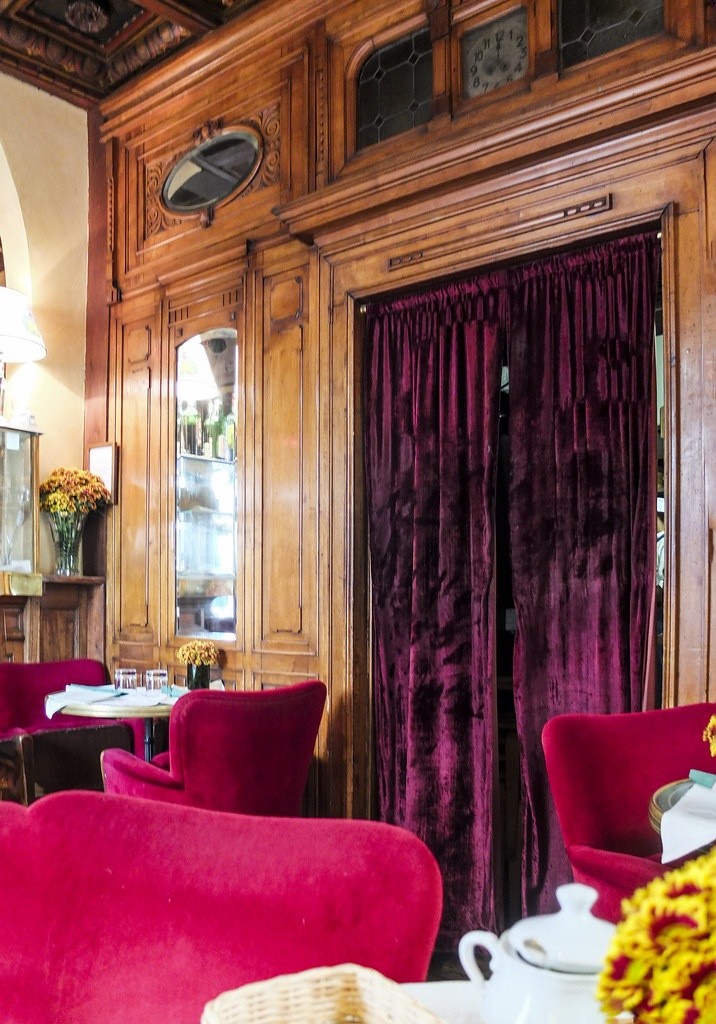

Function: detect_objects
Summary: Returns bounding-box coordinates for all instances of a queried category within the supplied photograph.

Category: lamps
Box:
[0,285,49,413]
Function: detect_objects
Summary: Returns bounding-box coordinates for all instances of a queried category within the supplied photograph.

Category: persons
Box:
[656,491,667,588]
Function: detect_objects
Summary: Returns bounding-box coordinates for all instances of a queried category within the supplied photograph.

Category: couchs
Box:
[0,658,149,803]
[540,699,716,928]
[0,789,443,1024]
[100,680,328,819]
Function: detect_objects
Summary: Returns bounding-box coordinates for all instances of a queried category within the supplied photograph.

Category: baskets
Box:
[202,963,451,1023]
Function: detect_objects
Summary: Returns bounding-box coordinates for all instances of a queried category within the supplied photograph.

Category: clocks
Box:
[449,0,538,120]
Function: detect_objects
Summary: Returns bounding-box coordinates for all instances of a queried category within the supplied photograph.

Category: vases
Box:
[186,663,210,689]
[47,510,88,577]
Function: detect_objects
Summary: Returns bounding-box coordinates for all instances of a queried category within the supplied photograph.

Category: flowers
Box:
[177,640,221,666]
[38,466,113,568]
[597,844,716,1024]
[703,714,716,757]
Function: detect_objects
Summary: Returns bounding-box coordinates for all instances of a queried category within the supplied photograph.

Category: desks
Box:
[648,779,716,858]
[43,689,172,760]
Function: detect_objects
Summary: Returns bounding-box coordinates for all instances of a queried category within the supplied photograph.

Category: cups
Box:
[457,882,625,1024]
[145,669,168,699]
[114,666,137,694]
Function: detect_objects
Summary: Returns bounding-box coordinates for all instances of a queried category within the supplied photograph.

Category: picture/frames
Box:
[86,443,119,506]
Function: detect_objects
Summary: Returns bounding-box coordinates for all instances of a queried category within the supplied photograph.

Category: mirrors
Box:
[173,325,240,642]
[156,123,263,218]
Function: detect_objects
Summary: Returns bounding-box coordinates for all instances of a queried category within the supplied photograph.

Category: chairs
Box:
[13,720,135,806]
